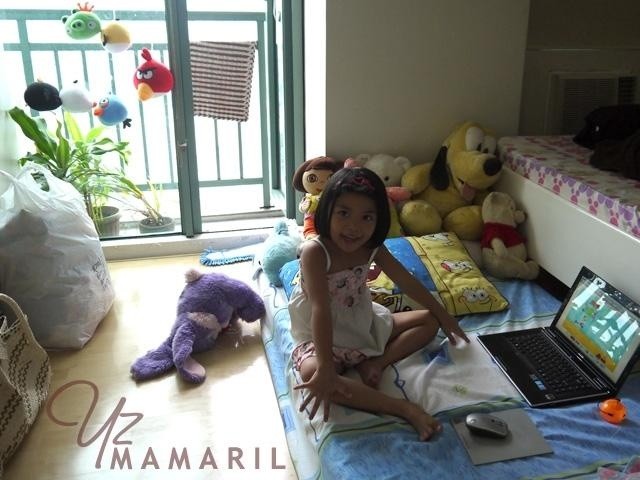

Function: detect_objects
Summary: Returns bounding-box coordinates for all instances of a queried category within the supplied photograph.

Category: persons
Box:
[251,120,540,293]
[286,165,469,441]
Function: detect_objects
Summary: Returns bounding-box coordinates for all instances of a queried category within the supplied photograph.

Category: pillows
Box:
[281,232,512,322]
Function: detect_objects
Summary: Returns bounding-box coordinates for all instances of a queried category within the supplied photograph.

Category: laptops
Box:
[476,265,640,408]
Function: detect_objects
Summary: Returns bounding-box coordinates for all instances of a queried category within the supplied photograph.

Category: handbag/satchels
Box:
[0,293,53,478]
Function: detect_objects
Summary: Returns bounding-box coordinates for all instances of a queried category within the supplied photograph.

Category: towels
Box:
[188,40,257,123]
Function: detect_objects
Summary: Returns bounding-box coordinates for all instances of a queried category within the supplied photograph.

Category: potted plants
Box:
[9,106,175,238]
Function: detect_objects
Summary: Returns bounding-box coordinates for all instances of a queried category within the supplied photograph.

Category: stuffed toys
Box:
[24,79,62,112]
[61,2,101,42]
[133,47,174,101]
[93,90,133,128]
[595,396,627,425]
[128,269,265,387]
[100,21,132,52]
[58,81,97,114]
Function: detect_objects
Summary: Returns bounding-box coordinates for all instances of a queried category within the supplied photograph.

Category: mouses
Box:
[465,413,508,439]
